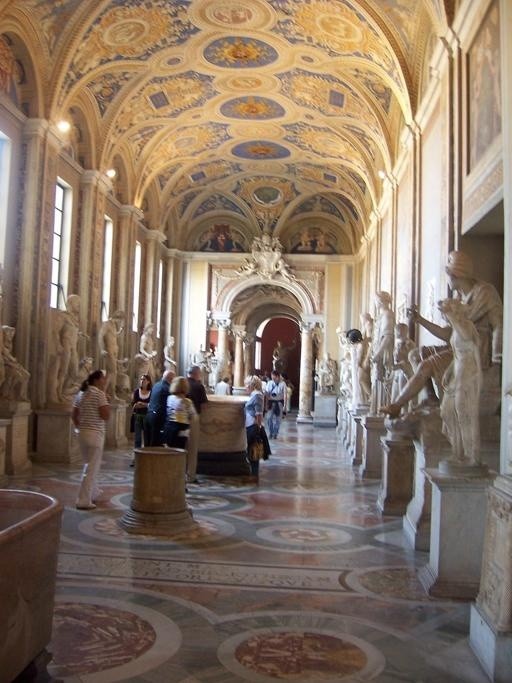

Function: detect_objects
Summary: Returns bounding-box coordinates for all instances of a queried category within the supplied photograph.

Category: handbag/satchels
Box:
[248,438,265,462]
[130,413,136,433]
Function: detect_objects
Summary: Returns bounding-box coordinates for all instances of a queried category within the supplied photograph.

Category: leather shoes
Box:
[192,478,200,484]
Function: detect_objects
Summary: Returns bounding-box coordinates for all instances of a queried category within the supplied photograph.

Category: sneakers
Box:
[273,433,278,439]
[268,433,273,440]
[77,503,97,509]
[185,488,190,494]
[129,459,135,467]
[92,489,104,503]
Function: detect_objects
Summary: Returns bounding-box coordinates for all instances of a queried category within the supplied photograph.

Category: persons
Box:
[317,247,502,467]
[70,369,109,510]
[272,337,297,373]
[0,294,213,413]
[129,367,294,483]
